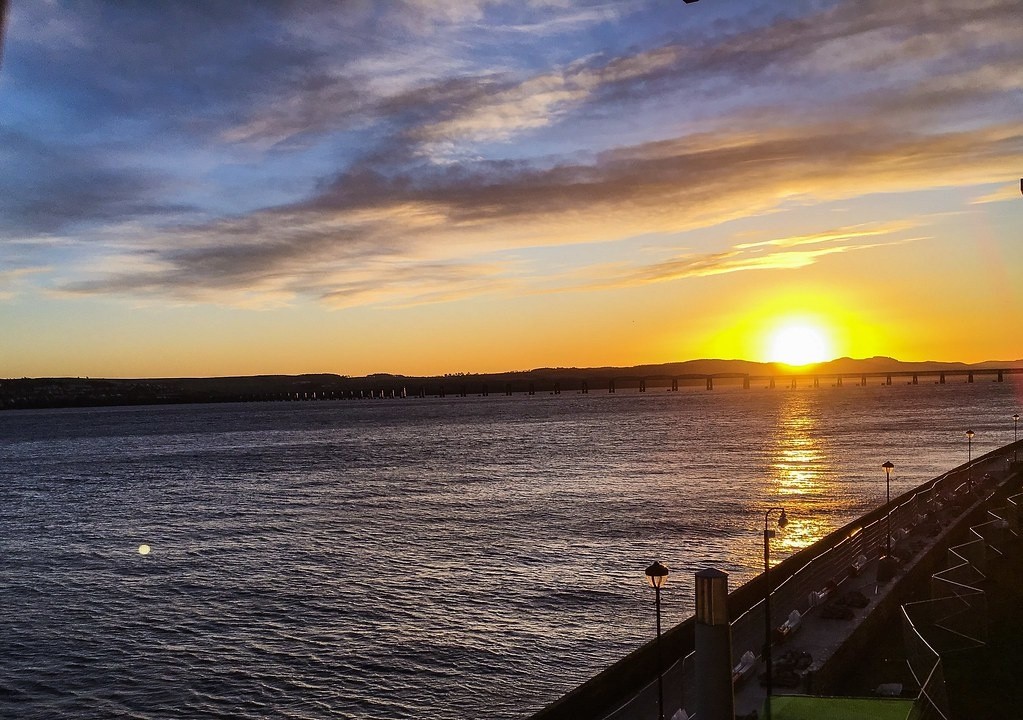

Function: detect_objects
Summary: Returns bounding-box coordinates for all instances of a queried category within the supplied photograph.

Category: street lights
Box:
[881,460,896,558]
[1011,413,1020,462]
[964,430,975,495]
[644,560,668,720]
[763,506,790,716]
